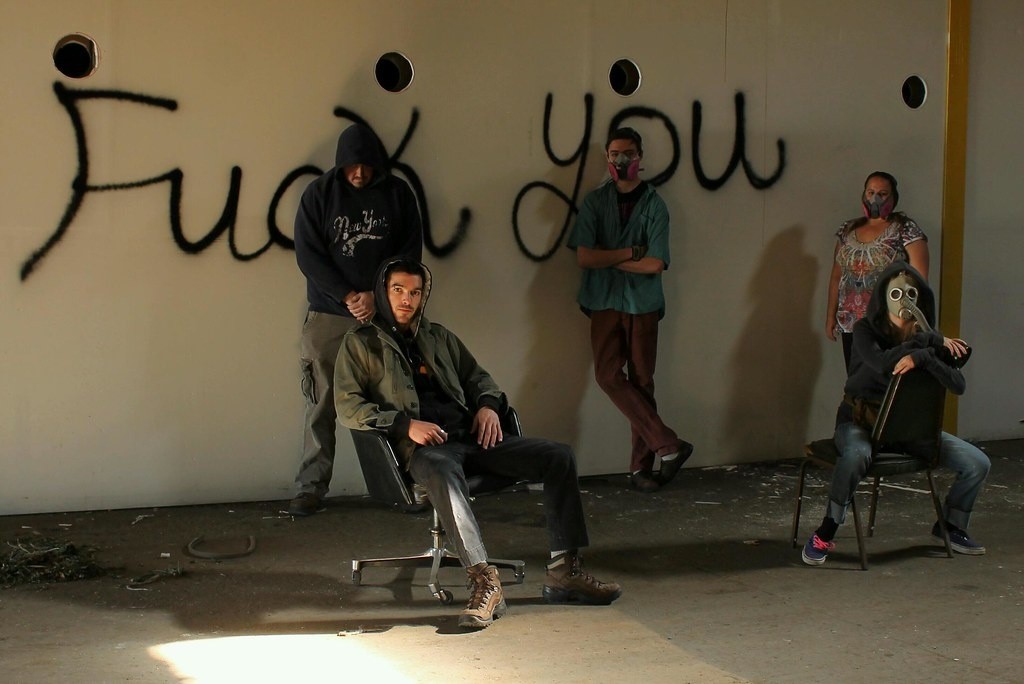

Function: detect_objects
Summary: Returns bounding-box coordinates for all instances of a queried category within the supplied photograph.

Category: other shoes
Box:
[628,470,660,493]
[657,440,694,486]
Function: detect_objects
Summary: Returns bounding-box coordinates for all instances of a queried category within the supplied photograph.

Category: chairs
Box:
[789,367,955,572]
[350,406,526,607]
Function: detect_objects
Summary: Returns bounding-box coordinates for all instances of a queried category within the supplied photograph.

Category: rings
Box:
[439,431,444,435]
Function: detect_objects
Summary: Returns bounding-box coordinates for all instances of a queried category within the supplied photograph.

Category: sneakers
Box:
[931,522,986,554]
[802,531,835,565]
[288,493,322,515]
[457,563,508,627]
[542,552,623,604]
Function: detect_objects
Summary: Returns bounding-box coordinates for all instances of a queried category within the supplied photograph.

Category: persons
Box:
[565,126,695,493]
[825,173,929,380]
[284,123,427,517]
[802,261,990,564]
[334,254,620,627]
